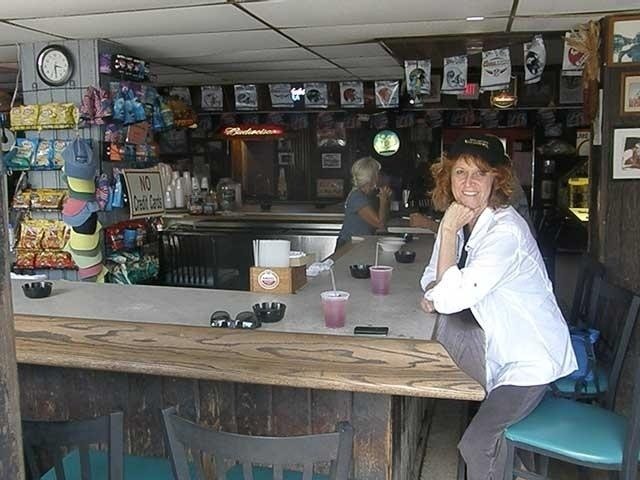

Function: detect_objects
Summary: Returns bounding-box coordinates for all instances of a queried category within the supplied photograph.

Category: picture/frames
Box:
[610,125,640,183]
[319,152,342,170]
[617,69,639,118]
[605,17,640,67]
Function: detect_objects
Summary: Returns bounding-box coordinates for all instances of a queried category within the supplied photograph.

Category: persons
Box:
[408,185,538,239]
[334,157,393,253]
[418,128,580,480]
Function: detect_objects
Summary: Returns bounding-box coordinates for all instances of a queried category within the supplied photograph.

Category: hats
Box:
[449,134,504,164]
[62,138,108,283]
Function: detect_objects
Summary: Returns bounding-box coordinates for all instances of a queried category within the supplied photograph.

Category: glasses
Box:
[210,311,261,328]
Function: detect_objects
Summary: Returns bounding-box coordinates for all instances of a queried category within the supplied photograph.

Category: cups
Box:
[369,265,395,293]
[320,291,350,330]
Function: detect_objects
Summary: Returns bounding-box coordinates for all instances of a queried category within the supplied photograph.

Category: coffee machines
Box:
[398,183,421,217]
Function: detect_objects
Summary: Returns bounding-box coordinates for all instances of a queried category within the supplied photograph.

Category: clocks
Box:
[38,43,74,86]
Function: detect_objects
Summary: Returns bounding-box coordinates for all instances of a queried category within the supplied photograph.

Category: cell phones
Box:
[354,326,388,334]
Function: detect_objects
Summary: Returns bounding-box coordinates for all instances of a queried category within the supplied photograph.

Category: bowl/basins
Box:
[231,312,260,329]
[165,163,236,217]
[376,236,416,263]
[210,309,232,328]
[23,282,53,297]
[348,262,370,278]
[252,301,286,322]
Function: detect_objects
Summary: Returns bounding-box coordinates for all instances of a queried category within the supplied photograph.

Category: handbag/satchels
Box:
[570,329,594,381]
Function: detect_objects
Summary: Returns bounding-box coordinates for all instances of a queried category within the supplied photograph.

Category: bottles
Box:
[278,165,288,201]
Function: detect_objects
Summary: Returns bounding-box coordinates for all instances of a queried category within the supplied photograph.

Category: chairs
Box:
[506,360,640,480]
[553,253,603,330]
[160,403,356,480]
[153,230,239,291]
[550,274,639,410]
[20,410,191,479]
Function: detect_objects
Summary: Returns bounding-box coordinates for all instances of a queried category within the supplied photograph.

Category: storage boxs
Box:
[248,263,306,293]
